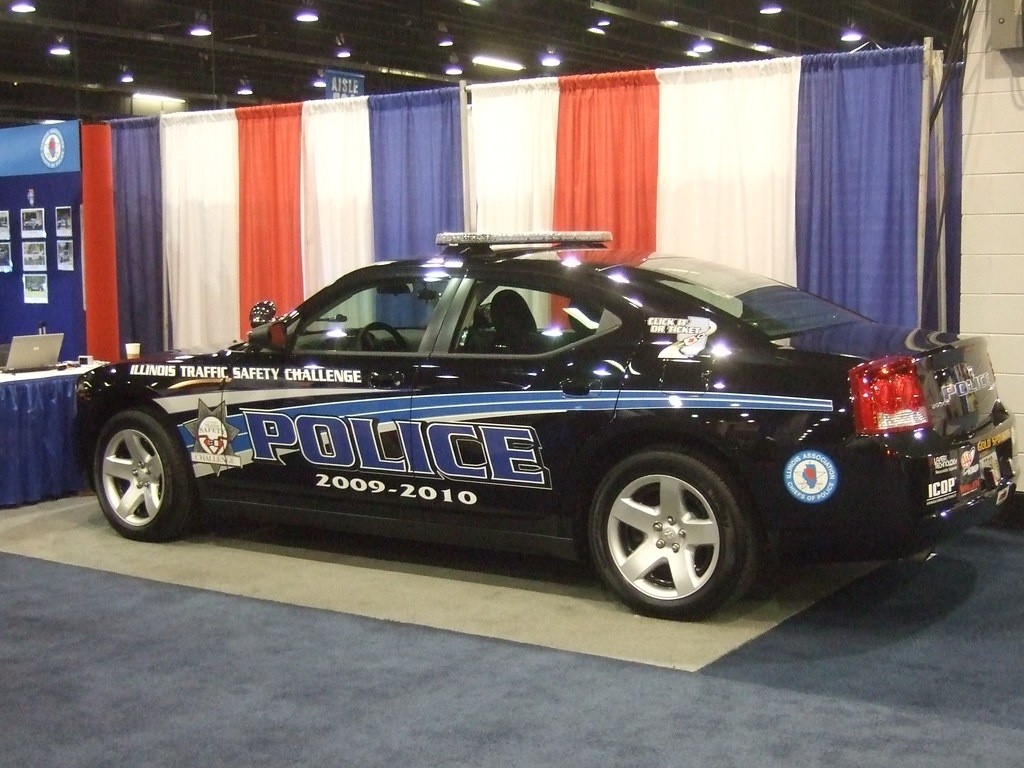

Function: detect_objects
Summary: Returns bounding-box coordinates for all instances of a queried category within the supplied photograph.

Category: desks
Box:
[1,360,111,507]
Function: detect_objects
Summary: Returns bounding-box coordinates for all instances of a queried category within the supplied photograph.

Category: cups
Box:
[125,343,141,359]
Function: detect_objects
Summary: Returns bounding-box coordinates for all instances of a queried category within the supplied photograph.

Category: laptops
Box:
[0,333,64,373]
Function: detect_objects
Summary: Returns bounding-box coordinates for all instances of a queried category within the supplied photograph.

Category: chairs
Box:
[553,299,603,347]
[489,289,539,354]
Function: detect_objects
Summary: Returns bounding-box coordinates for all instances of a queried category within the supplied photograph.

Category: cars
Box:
[70,230,1016,623]
